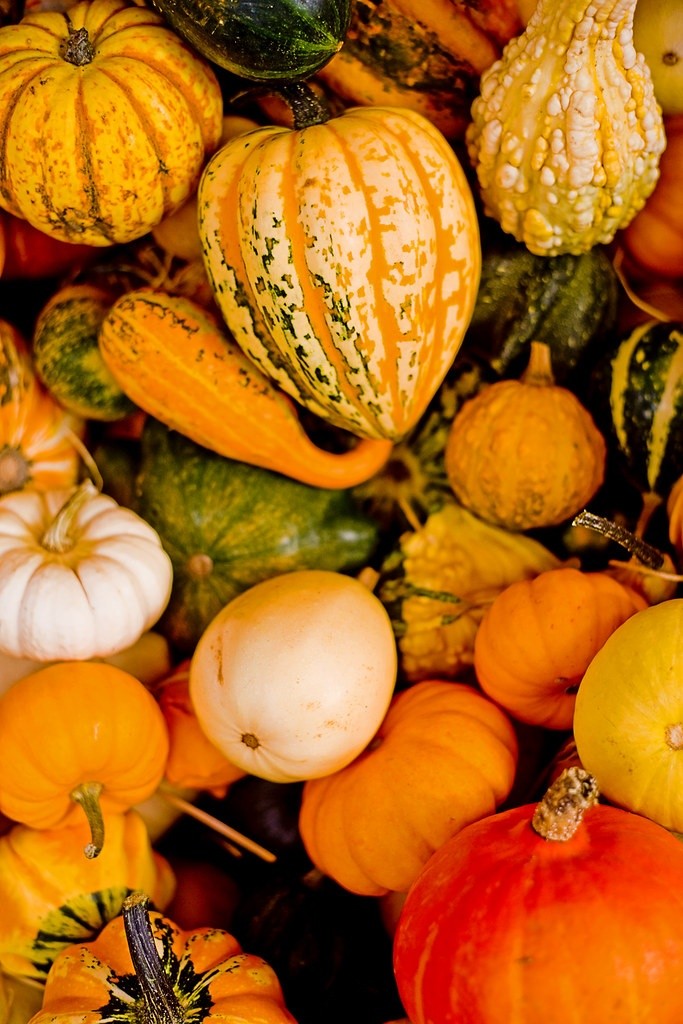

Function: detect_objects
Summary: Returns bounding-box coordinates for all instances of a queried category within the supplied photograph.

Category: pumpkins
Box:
[0,0,683,1024]
